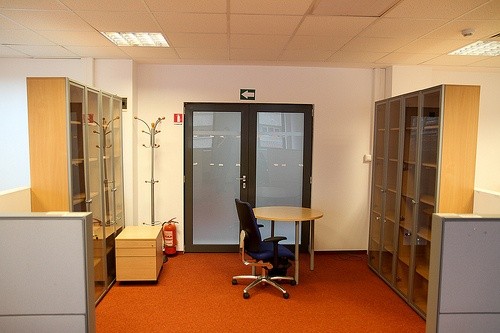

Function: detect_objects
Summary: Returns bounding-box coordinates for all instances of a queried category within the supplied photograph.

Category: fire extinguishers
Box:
[162,217,178,256]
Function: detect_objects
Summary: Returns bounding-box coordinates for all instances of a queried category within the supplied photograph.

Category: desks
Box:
[252,205,324,284]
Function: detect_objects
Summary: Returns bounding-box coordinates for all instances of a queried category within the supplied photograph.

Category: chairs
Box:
[232,199,297,299]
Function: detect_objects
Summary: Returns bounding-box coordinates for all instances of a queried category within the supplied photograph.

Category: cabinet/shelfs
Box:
[26,77,125,306]
[115,225,164,283]
[366,84,482,323]
